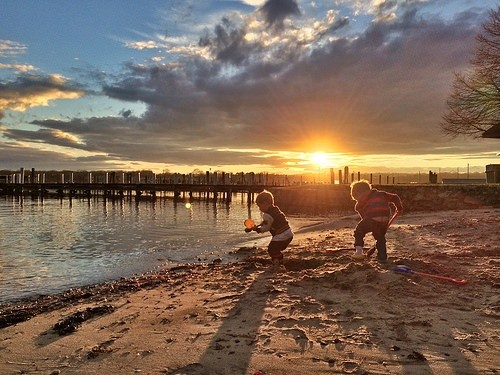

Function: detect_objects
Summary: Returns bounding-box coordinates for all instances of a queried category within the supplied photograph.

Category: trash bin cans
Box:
[485,164,500,183]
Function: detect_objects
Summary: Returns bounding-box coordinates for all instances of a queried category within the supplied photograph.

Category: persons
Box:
[350,179,402,264]
[245,190,294,263]
